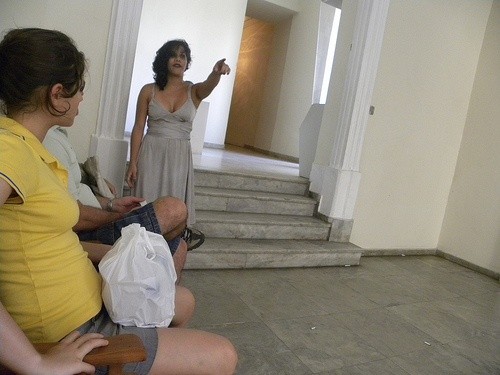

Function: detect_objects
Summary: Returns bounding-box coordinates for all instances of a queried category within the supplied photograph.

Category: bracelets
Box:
[107,199,112,211]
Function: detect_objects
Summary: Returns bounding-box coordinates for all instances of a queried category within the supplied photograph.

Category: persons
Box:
[126,41,231,226]
[0,29,238,375]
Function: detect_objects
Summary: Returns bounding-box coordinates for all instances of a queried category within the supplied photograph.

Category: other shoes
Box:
[179,225,204,251]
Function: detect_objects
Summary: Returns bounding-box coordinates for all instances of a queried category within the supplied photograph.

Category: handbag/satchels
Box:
[79,156,114,200]
[97,224,179,329]
